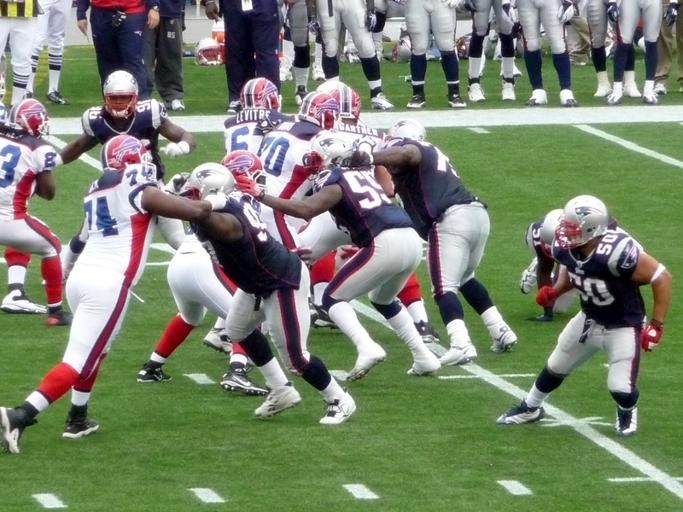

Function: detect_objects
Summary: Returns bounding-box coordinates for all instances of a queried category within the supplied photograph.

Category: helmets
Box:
[195,38,224,65]
[8,98,50,139]
[540,195,609,258]
[100,135,153,172]
[103,70,139,120]
[179,149,267,203]
[237,77,427,181]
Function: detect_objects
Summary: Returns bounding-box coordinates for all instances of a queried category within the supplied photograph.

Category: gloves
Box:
[558,0,574,23]
[528,313,553,321]
[159,143,183,158]
[607,3,619,23]
[536,286,559,308]
[640,319,663,352]
[365,10,377,31]
[663,3,679,27]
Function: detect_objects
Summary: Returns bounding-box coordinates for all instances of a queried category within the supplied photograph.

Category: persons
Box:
[139,150,356,425]
[497,194,671,436]
[1,98,73,325]
[201,0,683,112]
[204,78,517,380]
[1,0,188,111]
[40,70,196,285]
[1,135,227,454]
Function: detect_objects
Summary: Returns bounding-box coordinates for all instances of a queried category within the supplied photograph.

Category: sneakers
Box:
[47,91,67,105]
[183,50,193,56]
[312,66,326,81]
[496,400,545,425]
[467,56,522,103]
[62,417,100,439]
[560,89,578,106]
[489,323,518,352]
[407,320,478,377]
[279,67,293,81]
[0,407,38,454]
[519,257,538,294]
[570,37,667,105]
[615,405,637,437]
[524,89,548,105]
[137,363,173,382]
[203,298,387,424]
[0,274,74,326]
[164,98,185,110]
[448,94,467,108]
[227,100,241,113]
